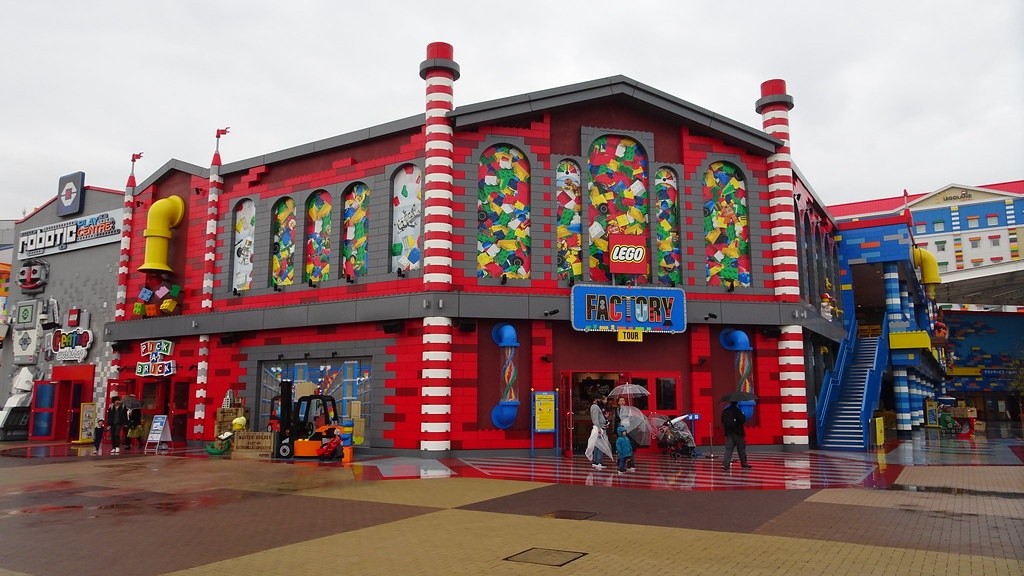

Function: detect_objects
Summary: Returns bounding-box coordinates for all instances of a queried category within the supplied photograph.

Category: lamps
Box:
[346,274,354,284]
[501,274,506,285]
[274,283,281,292]
[422,299,429,309]
[801,310,808,319]
[191,320,198,327]
[309,279,316,288]
[136,201,144,208]
[397,268,405,278]
[438,299,443,309]
[105,328,111,334]
[233,288,240,296]
[728,284,734,292]
[794,193,828,226]
[569,277,574,287]
[793,310,798,318]
[194,188,202,195]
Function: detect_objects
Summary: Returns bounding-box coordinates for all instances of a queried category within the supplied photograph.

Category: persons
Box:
[820,293,835,322]
[721,401,752,470]
[91,394,141,454]
[589,395,638,474]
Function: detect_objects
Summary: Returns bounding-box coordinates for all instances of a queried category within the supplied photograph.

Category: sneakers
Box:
[592,463,607,468]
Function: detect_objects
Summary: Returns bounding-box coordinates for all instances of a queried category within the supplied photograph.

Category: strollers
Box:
[658,415,697,460]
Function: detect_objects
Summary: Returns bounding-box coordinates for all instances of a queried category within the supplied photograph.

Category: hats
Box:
[112,396,120,403]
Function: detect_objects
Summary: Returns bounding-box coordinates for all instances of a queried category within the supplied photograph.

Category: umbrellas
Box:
[717,392,759,401]
[120,397,144,410]
[648,414,691,442]
[607,382,650,406]
[619,406,653,446]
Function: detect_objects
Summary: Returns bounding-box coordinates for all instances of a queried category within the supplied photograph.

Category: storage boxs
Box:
[953,407,977,418]
[214,423,250,438]
[217,407,249,422]
[974,421,986,431]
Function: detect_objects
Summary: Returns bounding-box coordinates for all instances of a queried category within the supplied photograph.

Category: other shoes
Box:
[627,468,635,470]
[743,465,752,469]
[618,471,626,474]
[111,447,120,452]
[94,450,97,454]
[722,465,727,470]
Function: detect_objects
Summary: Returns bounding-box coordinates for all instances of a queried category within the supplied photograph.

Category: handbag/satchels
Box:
[127,425,143,437]
